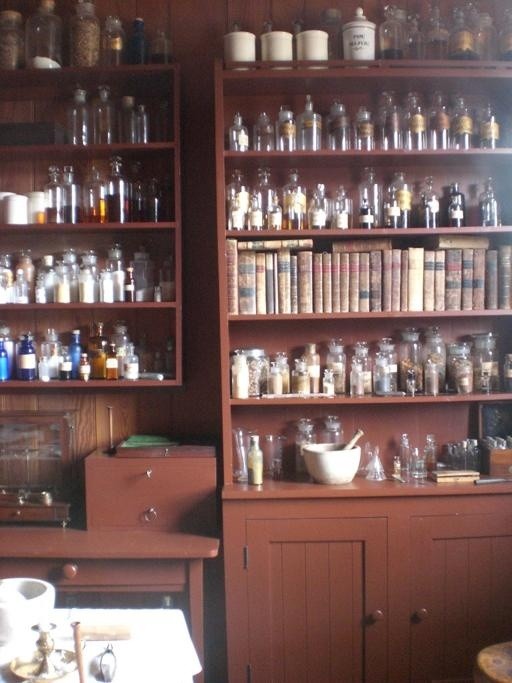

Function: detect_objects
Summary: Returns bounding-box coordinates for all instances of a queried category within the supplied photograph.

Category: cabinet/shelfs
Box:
[1,65,184,392]
[214,53,511,683]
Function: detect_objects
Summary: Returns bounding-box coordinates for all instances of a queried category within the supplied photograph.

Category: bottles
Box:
[226,163,504,228]
[226,84,512,151]
[1,322,176,382]
[5,151,177,225]
[3,1,176,66]
[230,415,511,486]
[228,320,512,395]
[48,72,176,146]
[223,3,512,72]
[0,242,175,303]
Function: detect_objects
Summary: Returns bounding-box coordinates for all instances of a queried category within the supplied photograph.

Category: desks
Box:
[1,521,222,683]
[30,605,203,683]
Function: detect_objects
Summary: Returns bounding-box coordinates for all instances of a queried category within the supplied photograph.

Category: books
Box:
[431,470,480,483]
[226,234,512,315]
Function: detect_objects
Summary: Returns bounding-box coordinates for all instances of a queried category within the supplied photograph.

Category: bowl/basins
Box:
[302,441,362,484]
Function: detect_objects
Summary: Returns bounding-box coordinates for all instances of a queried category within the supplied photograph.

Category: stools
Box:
[473,640,512,683]
